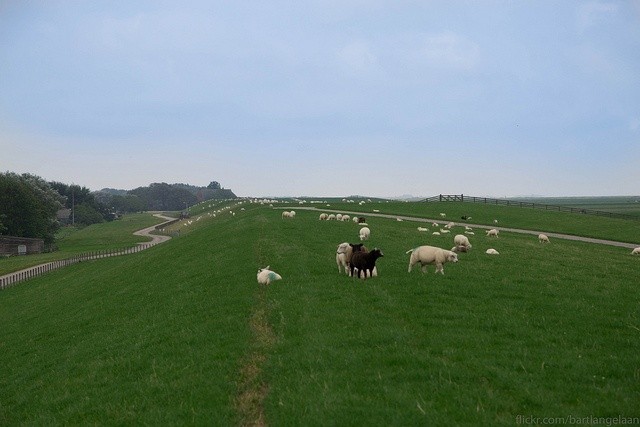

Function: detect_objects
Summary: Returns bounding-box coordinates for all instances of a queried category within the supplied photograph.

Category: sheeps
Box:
[336,213,342,220]
[373,208,499,238]
[358,217,365,222]
[290,210,296,215]
[352,216,359,222]
[486,248,499,255]
[341,214,350,221]
[538,233,550,243]
[327,214,335,220]
[406,246,459,276]
[282,211,292,217]
[319,212,328,220]
[352,248,384,279]
[359,227,371,241]
[454,234,472,249]
[257,264,282,285]
[349,243,368,278]
[336,242,353,277]
[630,246,640,255]
